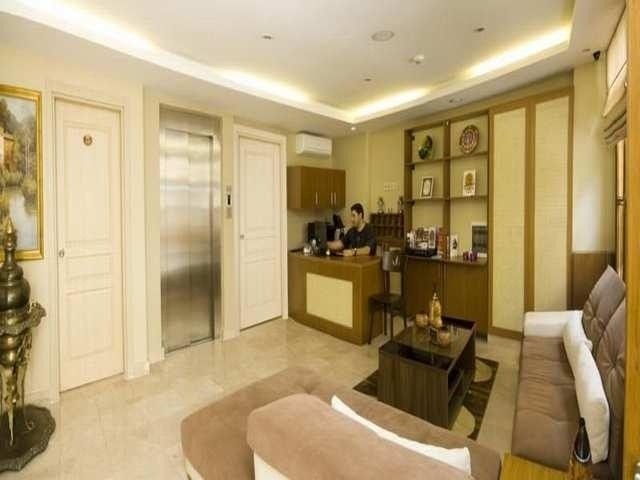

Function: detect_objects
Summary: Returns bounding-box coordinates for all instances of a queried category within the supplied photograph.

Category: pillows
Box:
[562,265,627,480]
[330,395,472,477]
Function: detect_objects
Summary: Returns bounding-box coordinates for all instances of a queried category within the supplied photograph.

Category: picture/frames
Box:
[419,176,434,198]
[1,83,45,264]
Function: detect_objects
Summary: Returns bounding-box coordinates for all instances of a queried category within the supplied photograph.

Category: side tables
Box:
[500,452,598,479]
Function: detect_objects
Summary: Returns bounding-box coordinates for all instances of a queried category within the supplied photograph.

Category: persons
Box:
[327,203,377,257]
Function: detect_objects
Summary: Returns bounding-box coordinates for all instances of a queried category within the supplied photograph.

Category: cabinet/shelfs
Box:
[402,108,493,343]
[287,164,345,211]
[366,212,404,247]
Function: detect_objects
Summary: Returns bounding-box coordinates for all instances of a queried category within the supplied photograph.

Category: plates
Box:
[459,125,481,155]
[417,134,433,159]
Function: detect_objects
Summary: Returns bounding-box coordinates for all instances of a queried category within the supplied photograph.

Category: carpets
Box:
[352,354,499,442]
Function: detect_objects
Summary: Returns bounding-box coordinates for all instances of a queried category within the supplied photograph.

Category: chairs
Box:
[369,250,407,343]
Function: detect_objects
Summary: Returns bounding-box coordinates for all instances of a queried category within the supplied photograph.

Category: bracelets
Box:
[352,247,357,256]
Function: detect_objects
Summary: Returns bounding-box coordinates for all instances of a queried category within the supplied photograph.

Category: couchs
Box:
[510,265,627,480]
[181,365,502,479]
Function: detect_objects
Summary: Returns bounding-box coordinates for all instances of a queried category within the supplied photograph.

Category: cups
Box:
[414,311,430,332]
[437,326,452,349]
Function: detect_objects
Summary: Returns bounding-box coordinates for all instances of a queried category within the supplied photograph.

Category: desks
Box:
[287,249,383,346]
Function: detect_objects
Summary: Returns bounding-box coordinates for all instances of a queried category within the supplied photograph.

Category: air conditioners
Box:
[296,133,332,159]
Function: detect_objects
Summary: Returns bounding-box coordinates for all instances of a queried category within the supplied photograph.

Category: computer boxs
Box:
[309,223,334,250]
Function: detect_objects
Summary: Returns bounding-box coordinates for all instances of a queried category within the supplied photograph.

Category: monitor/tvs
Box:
[315,221,327,256]
